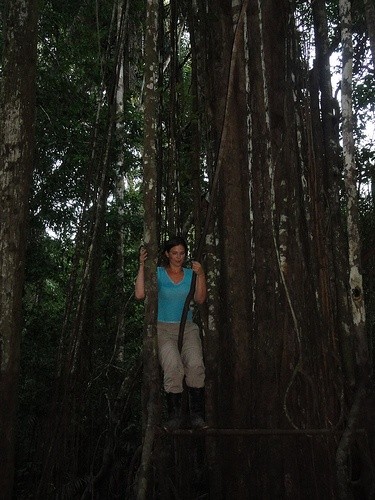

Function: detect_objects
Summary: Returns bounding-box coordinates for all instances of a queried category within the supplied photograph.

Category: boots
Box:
[189,388,207,428]
[167,393,184,428]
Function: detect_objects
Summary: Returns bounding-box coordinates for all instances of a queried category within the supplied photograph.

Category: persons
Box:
[135,237,206,429]
